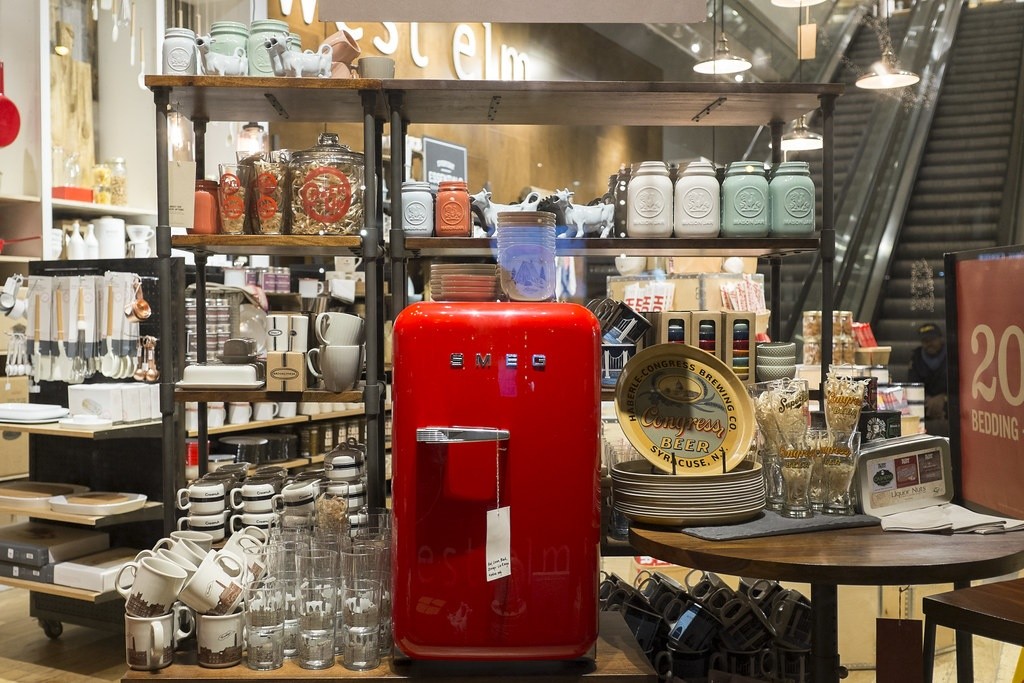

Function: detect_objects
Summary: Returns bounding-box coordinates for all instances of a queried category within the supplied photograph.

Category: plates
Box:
[668,322,749,382]
[216,352,263,364]
[0,480,148,515]
[183,428,299,480]
[610,342,765,527]
[429,210,557,302]
[0,403,69,423]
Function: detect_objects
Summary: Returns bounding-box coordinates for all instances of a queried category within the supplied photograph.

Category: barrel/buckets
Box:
[90,216,126,259]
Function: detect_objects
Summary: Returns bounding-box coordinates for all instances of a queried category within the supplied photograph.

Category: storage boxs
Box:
[637,311,755,389]
[68,382,163,427]
[266,350,308,392]
[0,522,143,594]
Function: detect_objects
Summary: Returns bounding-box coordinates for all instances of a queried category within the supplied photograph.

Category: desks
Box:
[628,516,1023,683]
[120,610,658,683]
[922,576,1024,683]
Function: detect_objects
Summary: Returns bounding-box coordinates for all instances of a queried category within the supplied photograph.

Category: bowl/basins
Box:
[52,229,62,260]
[757,341,796,382]
[615,257,648,276]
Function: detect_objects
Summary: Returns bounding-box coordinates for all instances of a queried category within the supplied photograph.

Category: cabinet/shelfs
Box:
[382,79,845,412]
[0,256,390,639]
[0,0,266,482]
[143,74,388,537]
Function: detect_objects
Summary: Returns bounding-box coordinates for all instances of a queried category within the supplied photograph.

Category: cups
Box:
[334,256,362,273]
[299,278,324,299]
[185,400,365,431]
[598,569,812,683]
[318,30,395,79]
[216,161,290,235]
[305,312,365,393]
[746,377,866,520]
[113,443,392,672]
[265,314,308,353]
[126,224,155,258]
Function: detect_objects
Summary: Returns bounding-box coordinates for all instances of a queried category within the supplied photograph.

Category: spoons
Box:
[33,284,161,384]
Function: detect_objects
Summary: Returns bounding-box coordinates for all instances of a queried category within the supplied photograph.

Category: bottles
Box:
[402,181,472,238]
[50,146,128,205]
[289,132,364,237]
[186,179,221,234]
[800,309,855,364]
[67,222,98,260]
[161,20,302,78]
[604,160,816,239]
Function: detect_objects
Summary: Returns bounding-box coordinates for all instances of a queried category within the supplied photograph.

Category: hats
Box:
[918,322,941,340]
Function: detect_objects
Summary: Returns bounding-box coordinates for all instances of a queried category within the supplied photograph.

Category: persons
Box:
[906,322,950,436]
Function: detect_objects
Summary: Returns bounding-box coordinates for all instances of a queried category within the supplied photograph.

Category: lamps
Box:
[854,0,921,89]
[768,1,823,151]
[693,0,752,74]
